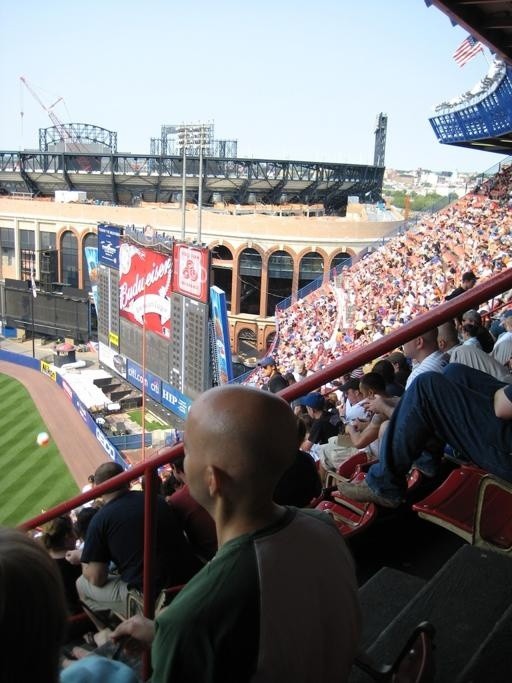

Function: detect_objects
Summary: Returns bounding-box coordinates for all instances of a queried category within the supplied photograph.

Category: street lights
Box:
[176,124,212,244]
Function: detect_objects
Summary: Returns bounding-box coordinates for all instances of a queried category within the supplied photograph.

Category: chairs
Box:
[62,447,512,683]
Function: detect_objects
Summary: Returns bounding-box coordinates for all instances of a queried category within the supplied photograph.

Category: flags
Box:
[453,35,482,68]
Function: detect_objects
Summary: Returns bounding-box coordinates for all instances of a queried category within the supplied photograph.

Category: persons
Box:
[0,165,512,683]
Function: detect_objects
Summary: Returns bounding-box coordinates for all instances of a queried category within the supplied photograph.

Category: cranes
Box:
[19,76,101,174]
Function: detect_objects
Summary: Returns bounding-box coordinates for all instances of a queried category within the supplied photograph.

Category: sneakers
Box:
[336,478,406,508]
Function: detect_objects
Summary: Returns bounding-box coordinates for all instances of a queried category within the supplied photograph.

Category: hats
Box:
[338,378,360,391]
[294,361,305,374]
[300,392,325,410]
[257,356,276,368]
[463,272,481,281]
